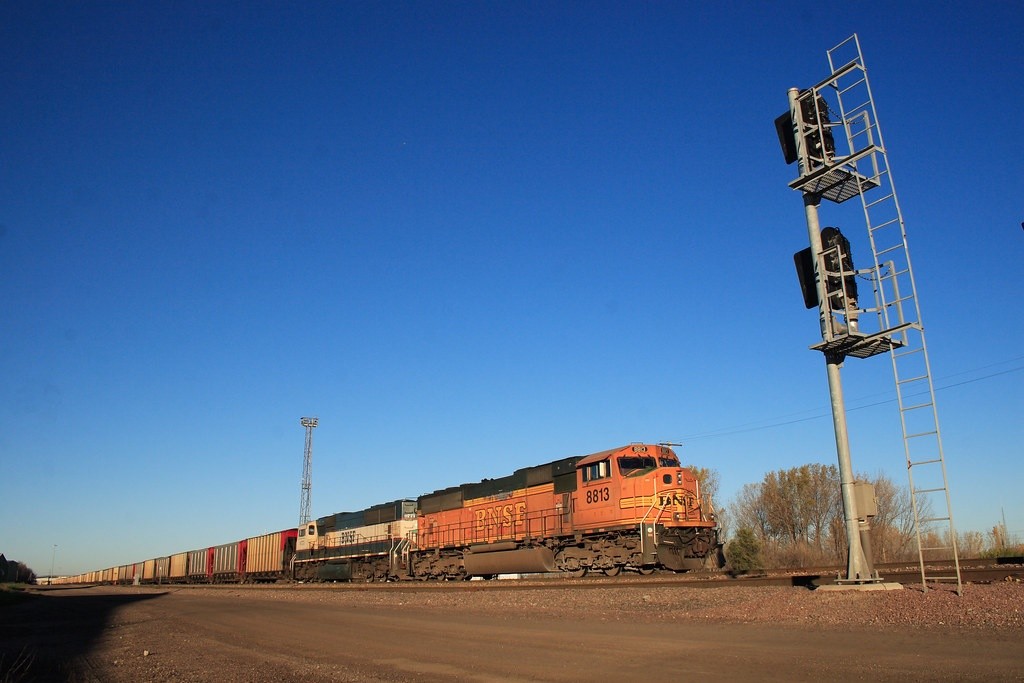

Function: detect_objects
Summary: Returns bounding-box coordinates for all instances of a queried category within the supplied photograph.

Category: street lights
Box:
[50,544,57,586]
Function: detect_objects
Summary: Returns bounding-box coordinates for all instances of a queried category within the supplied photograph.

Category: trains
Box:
[34,441,725,586]
